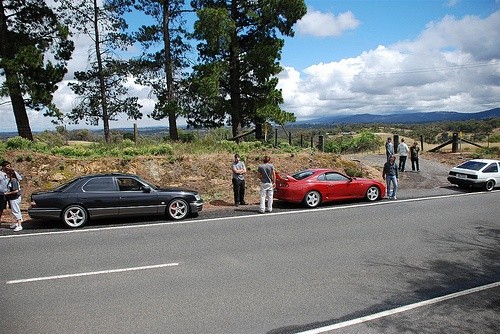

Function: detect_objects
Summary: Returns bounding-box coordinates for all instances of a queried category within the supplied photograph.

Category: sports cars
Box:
[272,169,386,208]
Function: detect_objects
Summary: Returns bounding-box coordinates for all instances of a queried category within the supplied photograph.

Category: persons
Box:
[397,138,410,173]
[256,155,277,214]
[0,160,25,232]
[409,141,422,173]
[382,154,399,200]
[384,137,394,162]
[230,153,249,207]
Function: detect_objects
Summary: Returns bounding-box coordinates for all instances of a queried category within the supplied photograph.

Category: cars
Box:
[28,174,204,228]
[447,159,500,191]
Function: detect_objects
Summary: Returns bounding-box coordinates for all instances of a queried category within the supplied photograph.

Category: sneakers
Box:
[10,224,18,228]
[14,226,23,231]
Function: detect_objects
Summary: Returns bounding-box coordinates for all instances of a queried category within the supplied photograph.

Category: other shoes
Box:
[236,203,239,206]
[258,211,263,213]
[393,196,397,200]
[418,170,421,172]
[241,202,248,205]
[412,171,415,172]
[266,209,271,212]
